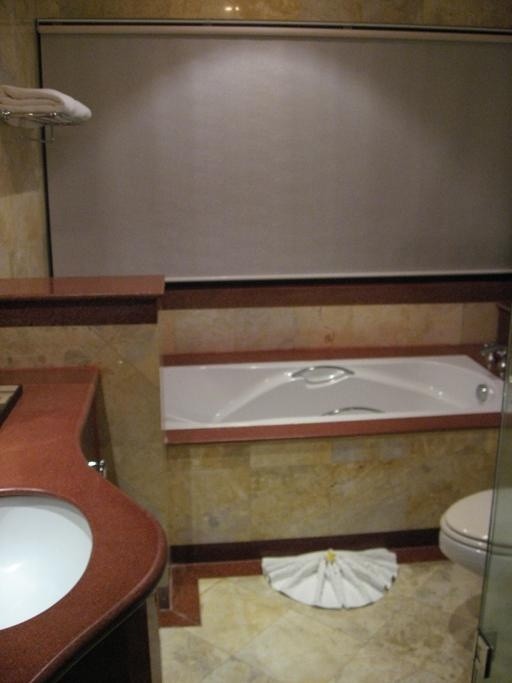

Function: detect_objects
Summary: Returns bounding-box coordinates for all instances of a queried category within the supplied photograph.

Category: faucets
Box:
[480,344,507,379]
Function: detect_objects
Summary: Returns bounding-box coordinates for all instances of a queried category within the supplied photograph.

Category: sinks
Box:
[1,488,94,631]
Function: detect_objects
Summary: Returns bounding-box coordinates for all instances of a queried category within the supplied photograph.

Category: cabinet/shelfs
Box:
[80,404,109,480]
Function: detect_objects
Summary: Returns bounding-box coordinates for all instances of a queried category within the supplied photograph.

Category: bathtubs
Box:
[159,355,511,429]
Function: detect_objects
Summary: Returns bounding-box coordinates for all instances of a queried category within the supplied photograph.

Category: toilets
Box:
[439,487,511,578]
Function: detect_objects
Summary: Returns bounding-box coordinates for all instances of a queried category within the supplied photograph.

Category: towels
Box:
[261,547,400,609]
[0,83,91,123]
